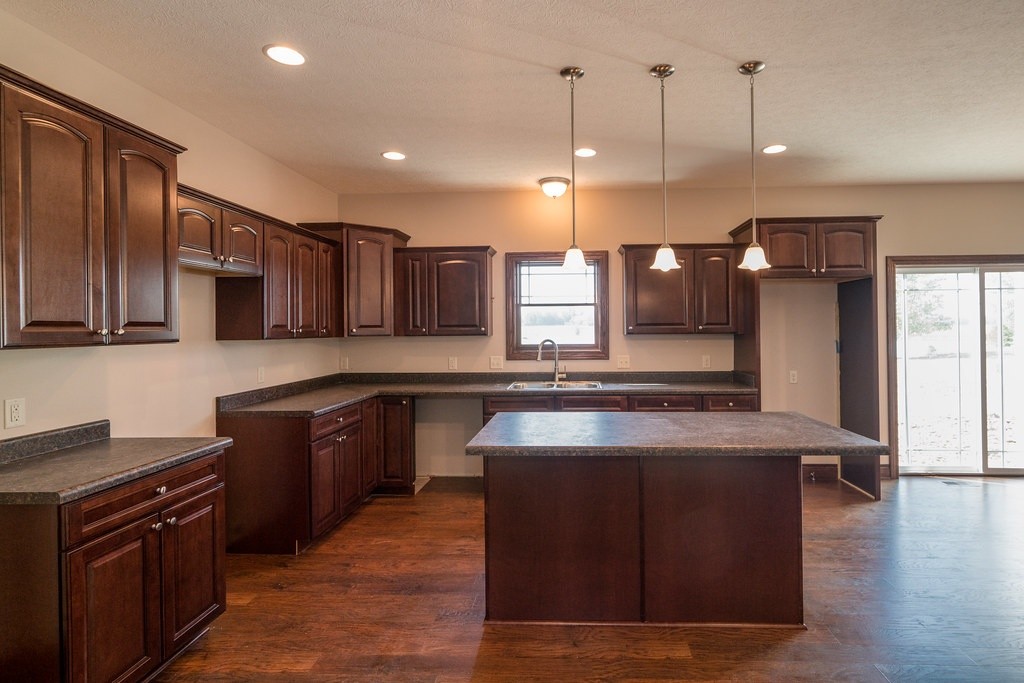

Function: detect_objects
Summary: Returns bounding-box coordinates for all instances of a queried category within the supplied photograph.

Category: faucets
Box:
[537,339,567,381]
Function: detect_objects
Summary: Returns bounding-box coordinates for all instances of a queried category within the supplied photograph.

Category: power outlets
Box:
[448,356,457,370]
[789,370,797,384]
[4,397,26,429]
[703,355,711,368]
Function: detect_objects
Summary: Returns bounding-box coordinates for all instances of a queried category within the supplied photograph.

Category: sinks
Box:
[556,381,601,390]
[506,380,554,390]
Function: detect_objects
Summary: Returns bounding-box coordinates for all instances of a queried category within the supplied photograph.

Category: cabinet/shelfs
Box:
[0,61,190,350]
[0,451,227,683]
[217,395,416,556]
[478,457,807,629]
[319,234,341,338]
[216,217,319,340]
[618,241,756,336]
[297,221,411,339]
[481,393,761,426]
[392,245,498,337]
[727,214,884,283]
[178,183,264,277]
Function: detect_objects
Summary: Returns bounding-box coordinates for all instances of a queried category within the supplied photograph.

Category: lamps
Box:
[538,178,571,199]
[560,68,589,276]
[649,64,682,272]
[737,60,773,270]
